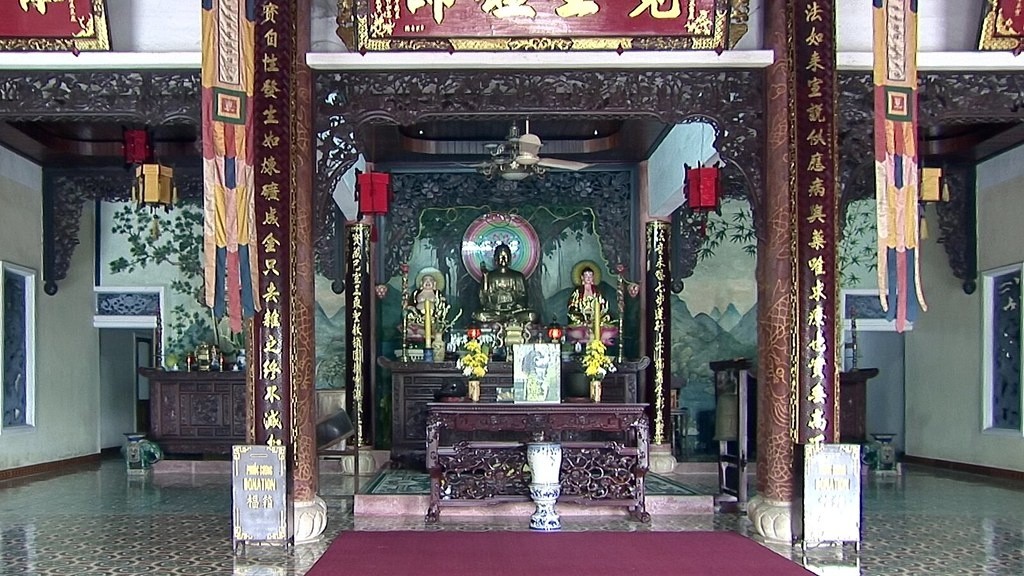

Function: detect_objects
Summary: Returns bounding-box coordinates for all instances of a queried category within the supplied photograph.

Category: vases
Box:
[468,381,480,403]
[589,381,602,402]
[432,332,445,363]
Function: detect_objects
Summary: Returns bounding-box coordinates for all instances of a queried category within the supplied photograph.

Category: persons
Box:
[567,266,615,325]
[404,274,452,339]
[472,242,541,327]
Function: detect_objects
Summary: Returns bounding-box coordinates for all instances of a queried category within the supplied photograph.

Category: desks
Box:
[710,360,879,502]
[423,398,652,523]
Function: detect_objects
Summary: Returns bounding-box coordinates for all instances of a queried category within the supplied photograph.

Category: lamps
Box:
[478,116,547,182]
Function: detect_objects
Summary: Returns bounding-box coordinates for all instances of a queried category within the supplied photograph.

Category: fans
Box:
[451,120,588,172]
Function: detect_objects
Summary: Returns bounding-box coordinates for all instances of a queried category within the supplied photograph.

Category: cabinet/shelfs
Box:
[377,352,650,470]
[314,389,346,461]
[140,366,246,456]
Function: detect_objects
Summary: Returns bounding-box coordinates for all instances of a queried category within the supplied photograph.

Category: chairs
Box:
[670,388,688,457]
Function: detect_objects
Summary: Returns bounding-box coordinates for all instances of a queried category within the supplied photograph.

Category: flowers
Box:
[582,341,617,380]
[455,340,489,380]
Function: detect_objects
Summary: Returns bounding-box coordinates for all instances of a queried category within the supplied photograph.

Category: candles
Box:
[424,298,431,349]
[594,299,600,341]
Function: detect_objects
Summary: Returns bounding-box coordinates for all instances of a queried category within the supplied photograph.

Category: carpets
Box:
[302,529,818,576]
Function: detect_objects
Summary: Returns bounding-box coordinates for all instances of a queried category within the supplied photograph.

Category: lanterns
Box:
[131,163,178,237]
[358,172,390,243]
[919,166,942,240]
[683,159,722,237]
[119,125,151,165]
